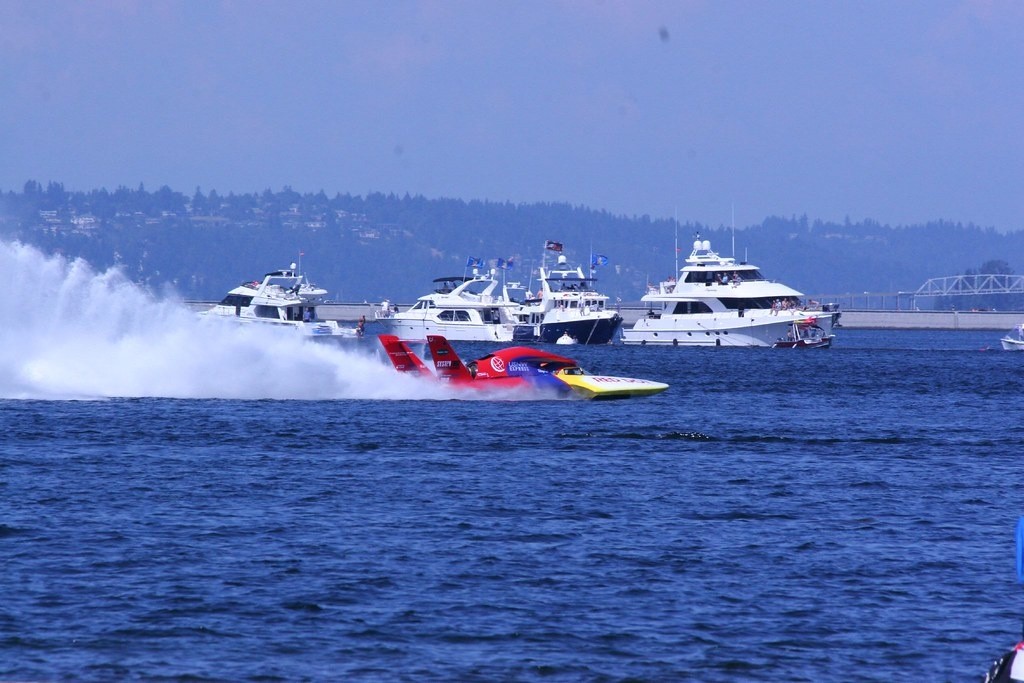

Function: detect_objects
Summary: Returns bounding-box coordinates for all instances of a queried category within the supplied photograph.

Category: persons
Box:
[394,304,398,314]
[788,330,792,341]
[381,300,390,318]
[645,277,676,293]
[716,271,738,286]
[358,315,365,337]
[607,339,613,344]
[537,288,543,305]
[493,310,499,323]
[561,283,578,292]
[768,299,819,317]
[303,307,311,323]
[442,283,448,293]
[525,288,531,307]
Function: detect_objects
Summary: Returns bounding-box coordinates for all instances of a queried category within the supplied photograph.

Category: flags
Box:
[495,258,514,271]
[465,256,483,269]
[590,254,608,266]
[544,240,564,252]
[300,250,304,256]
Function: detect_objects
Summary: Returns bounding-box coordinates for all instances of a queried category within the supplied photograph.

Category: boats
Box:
[373,237,624,348]
[377,330,670,405]
[619,205,837,348]
[196,252,370,341]
[1000,323,1024,351]
